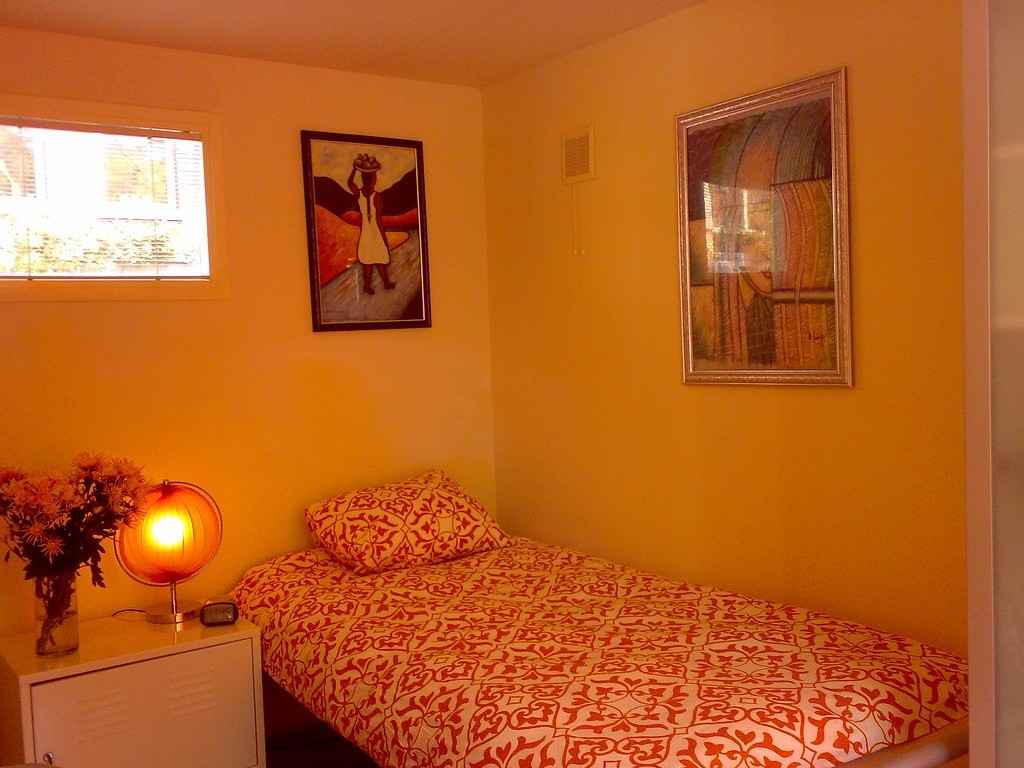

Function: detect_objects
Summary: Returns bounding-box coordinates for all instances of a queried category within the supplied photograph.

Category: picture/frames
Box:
[300,130,432,332]
[675,67,853,389]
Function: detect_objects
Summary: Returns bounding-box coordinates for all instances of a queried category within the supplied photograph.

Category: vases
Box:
[32,572,81,659]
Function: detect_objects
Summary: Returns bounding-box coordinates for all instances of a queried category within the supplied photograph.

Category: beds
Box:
[229,536,969,768]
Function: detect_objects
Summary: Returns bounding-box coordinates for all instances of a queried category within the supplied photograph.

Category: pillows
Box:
[304,470,518,574]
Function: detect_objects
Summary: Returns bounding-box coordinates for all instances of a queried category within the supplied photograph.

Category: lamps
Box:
[114,479,224,624]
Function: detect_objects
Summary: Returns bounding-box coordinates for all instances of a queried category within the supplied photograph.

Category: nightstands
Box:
[0,599,266,768]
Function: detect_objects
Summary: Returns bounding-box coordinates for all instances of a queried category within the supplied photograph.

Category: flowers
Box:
[0,450,156,651]
